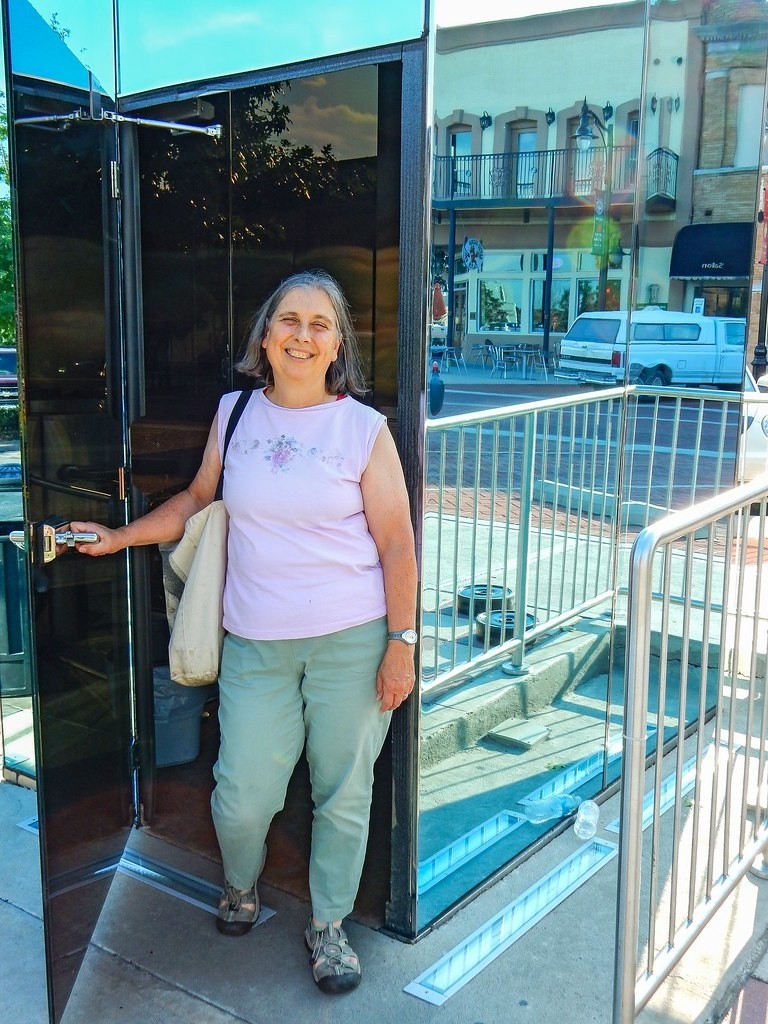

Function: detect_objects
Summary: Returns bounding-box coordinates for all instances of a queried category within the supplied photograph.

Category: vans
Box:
[552,303,748,404]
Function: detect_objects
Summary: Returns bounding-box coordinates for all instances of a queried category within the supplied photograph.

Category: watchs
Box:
[387,629,418,646]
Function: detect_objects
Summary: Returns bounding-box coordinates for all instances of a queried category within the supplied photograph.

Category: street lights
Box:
[571,95,614,311]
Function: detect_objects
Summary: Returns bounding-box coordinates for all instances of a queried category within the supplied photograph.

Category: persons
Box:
[71,274,419,994]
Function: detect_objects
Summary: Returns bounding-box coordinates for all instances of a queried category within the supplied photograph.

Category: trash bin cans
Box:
[152,655,207,768]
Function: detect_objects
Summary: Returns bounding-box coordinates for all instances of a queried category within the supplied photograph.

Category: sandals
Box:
[216,842,267,936]
[304,912,362,994]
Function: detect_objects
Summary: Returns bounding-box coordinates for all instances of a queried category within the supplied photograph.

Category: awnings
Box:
[669,223,755,280]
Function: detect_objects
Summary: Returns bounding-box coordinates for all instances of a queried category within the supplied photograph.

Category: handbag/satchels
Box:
[163,389,253,687]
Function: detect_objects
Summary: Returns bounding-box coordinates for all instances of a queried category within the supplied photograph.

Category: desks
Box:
[431,347,455,373]
[504,350,539,380]
[501,345,518,366]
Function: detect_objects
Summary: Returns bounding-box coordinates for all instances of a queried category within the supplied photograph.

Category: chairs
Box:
[428,337,560,382]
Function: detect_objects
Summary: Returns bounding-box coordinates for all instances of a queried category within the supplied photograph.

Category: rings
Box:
[403,694,408,698]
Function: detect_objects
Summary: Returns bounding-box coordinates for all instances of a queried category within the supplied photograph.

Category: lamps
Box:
[602,100,613,122]
[479,111,492,131]
[545,106,555,127]
